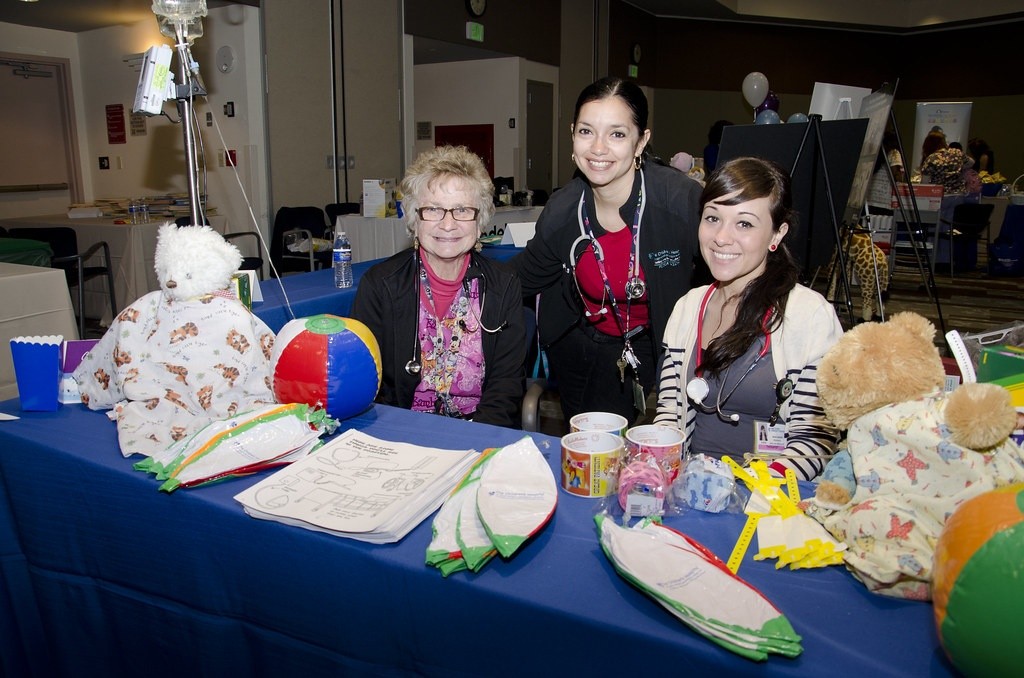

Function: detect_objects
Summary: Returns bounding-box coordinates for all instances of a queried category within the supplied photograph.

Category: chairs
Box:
[522,294,548,434]
[270,202,360,279]
[175,216,263,282]
[891,201,994,280]
[9,227,118,340]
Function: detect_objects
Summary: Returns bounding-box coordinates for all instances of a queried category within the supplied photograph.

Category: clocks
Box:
[632,42,641,65]
[465,0,487,18]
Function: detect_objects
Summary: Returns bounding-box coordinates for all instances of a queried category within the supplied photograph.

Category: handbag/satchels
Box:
[986,236,1023,277]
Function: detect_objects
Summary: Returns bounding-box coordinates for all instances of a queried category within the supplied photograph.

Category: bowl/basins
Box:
[624,424,687,482]
[559,430,626,499]
[569,412,629,439]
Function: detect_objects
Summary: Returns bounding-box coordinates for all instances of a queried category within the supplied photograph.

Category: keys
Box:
[617,358,627,383]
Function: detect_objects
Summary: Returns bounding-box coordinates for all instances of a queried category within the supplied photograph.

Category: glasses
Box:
[415,207,481,222]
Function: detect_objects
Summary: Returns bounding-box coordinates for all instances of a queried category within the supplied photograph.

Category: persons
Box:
[920,130,991,177]
[511,75,702,430]
[702,119,735,171]
[656,157,843,483]
[349,144,526,430]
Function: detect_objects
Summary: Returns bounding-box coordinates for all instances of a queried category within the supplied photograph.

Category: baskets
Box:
[1008,174,1024,206]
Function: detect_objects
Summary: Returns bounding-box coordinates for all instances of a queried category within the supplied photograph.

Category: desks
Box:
[981,190,1024,244]
[332,205,546,269]
[0,214,230,403]
[479,243,527,263]
[249,256,387,333]
[0,390,953,678]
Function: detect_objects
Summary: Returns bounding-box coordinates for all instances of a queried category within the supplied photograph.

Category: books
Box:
[65,193,218,218]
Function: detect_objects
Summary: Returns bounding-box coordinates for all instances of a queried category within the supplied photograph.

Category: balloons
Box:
[757,91,779,115]
[787,112,809,123]
[756,109,780,124]
[742,71,769,108]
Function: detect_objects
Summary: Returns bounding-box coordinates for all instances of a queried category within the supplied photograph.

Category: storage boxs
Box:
[9,335,64,412]
[231,273,251,312]
[57,339,101,404]
[362,178,397,218]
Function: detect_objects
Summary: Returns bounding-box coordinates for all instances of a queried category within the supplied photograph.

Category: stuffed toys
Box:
[73,221,278,458]
[815,310,1024,604]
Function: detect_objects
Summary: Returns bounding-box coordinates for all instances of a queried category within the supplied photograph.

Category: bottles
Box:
[139,196,150,224]
[333,232,353,289]
[129,197,140,225]
[526,196,532,207]
